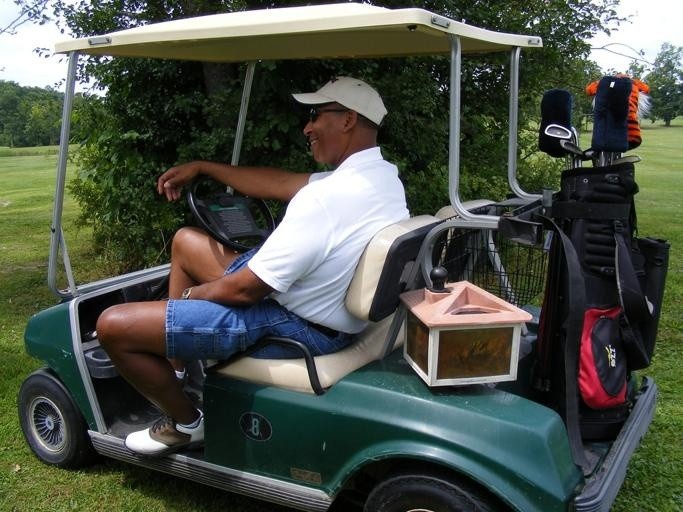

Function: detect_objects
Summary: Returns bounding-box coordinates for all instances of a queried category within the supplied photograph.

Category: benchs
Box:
[206,195,494,396]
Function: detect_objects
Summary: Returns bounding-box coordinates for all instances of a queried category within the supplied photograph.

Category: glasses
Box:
[310,105,349,123]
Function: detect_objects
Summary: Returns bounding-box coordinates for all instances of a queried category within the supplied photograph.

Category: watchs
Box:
[182,286,193,300]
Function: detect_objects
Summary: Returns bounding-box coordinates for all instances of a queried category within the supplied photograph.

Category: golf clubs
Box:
[545,124,643,170]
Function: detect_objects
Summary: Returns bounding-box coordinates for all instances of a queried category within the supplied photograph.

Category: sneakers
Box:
[124,407,205,456]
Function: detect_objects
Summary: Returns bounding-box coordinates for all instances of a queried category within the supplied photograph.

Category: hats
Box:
[290,76,388,126]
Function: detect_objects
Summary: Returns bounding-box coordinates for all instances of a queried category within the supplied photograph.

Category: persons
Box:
[96,76,411,456]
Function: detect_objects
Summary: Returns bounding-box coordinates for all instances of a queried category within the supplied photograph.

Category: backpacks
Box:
[517,162,674,445]
